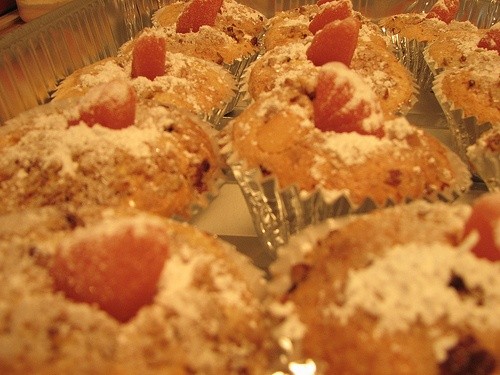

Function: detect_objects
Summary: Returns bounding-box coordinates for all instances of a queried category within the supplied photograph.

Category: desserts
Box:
[434,48,499,145]
[240,17,420,123]
[0,80,230,228]
[268,188,498,375]
[379,0,477,95]
[261,0,385,54]
[1,202,291,375]
[152,0,270,35]
[116,1,256,117]
[429,20,500,152]
[466,120,500,191]
[47,36,242,128]
[218,61,474,258]
[424,19,488,76]
